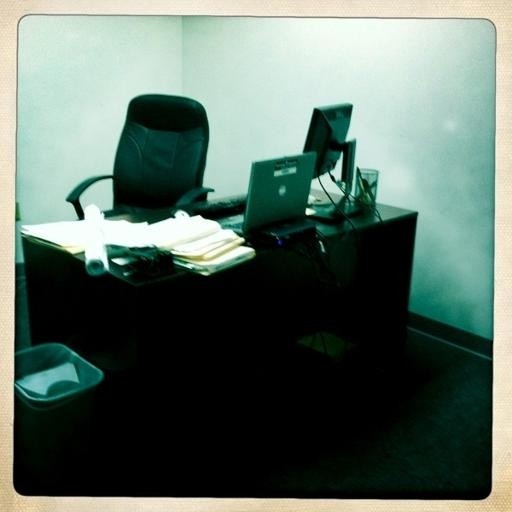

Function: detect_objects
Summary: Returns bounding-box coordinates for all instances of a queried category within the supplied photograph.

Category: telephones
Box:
[108,246,171,272]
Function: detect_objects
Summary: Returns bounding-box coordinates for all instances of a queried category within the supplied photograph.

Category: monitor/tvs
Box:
[303,101,363,222]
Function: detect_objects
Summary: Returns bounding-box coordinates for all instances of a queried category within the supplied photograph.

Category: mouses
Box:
[171,209,189,219]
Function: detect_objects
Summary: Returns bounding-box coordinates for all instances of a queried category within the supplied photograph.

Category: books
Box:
[139,215,256,278]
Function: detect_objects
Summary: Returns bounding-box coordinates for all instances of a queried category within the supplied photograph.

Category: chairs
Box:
[65,94,214,220]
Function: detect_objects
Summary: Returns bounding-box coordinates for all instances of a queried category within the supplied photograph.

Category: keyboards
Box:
[183,193,247,221]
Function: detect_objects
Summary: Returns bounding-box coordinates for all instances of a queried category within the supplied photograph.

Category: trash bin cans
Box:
[14,342,104,463]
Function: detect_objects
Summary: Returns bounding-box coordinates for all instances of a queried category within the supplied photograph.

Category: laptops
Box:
[212,150,317,237]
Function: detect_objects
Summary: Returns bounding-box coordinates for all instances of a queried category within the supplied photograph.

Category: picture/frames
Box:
[0,0,512,512]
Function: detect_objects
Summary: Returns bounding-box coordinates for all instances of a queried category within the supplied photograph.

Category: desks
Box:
[21,210,416,496]
